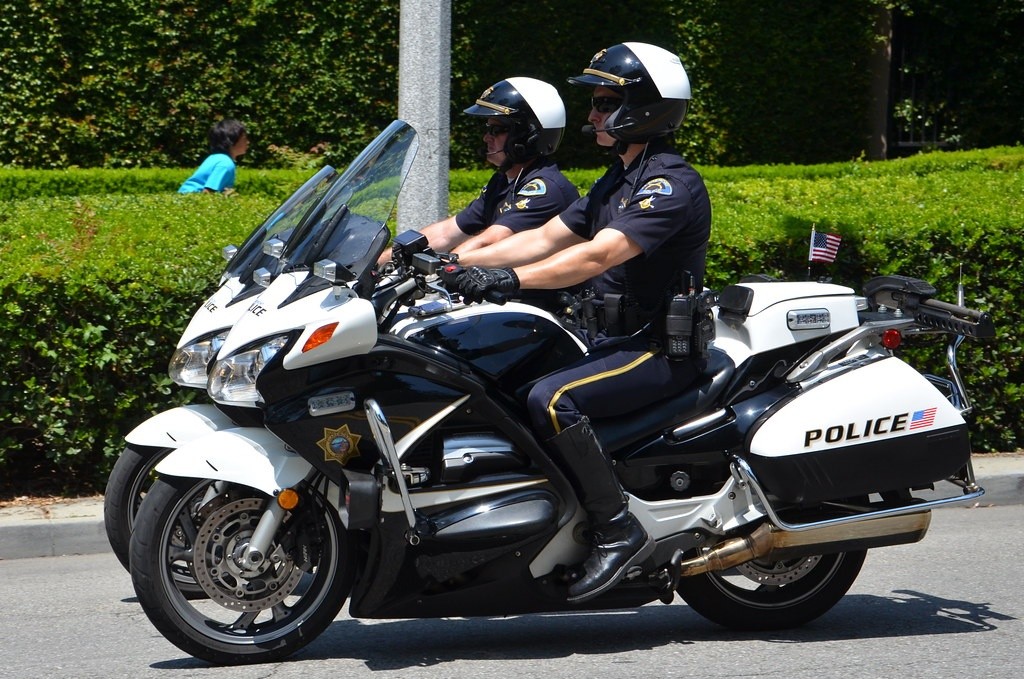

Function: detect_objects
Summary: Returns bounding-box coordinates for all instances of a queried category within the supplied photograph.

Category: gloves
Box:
[456,266,520,306]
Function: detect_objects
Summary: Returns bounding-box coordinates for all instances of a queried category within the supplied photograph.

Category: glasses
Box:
[592,96,623,113]
[484,122,515,136]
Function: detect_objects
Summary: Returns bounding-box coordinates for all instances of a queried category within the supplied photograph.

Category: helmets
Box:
[568,40,692,143]
[462,77,565,164]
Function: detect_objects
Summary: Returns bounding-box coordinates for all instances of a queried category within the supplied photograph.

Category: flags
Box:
[808,228,843,265]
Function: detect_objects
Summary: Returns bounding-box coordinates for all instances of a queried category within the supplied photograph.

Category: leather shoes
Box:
[567,511,656,603]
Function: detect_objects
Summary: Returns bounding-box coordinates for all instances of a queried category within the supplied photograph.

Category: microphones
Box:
[582,122,634,137]
[478,147,503,155]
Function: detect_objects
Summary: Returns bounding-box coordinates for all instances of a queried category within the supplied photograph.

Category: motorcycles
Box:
[100,120,995,666]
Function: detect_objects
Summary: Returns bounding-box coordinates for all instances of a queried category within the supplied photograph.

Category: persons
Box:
[439,40,712,605]
[177,118,251,197]
[371,77,584,318]
